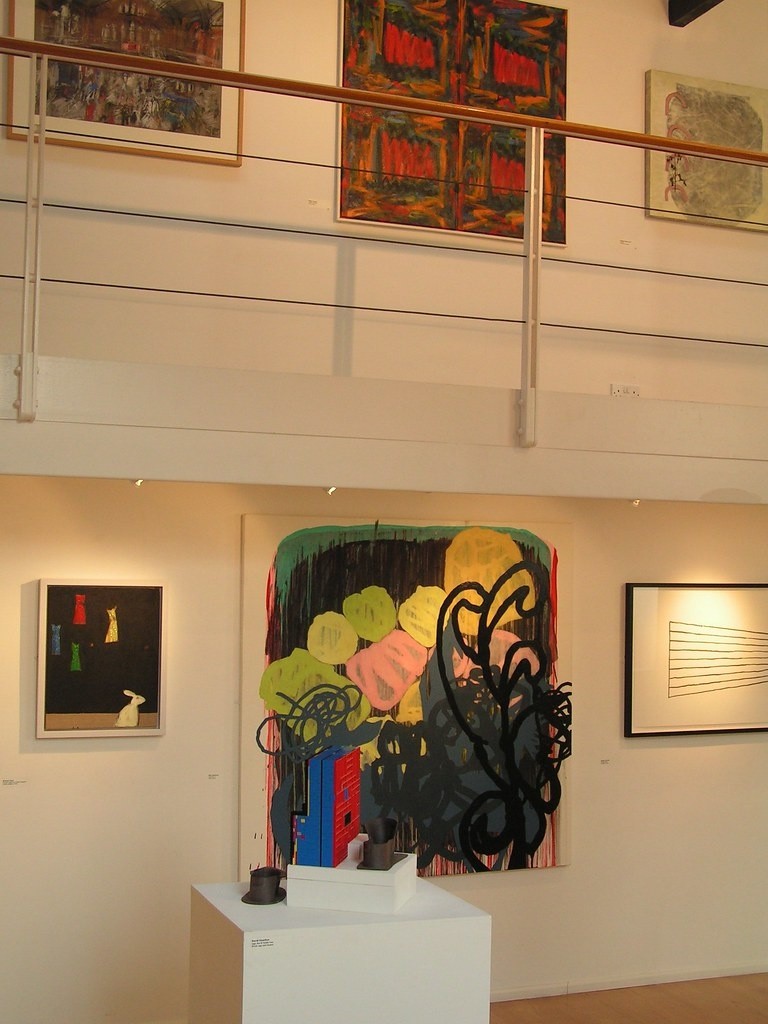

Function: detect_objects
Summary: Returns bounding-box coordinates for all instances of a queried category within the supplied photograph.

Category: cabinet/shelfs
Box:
[191,877,492,1023]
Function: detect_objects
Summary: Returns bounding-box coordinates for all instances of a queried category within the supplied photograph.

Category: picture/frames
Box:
[625,581,768,734]
[335,1,568,248]
[39,580,166,734]
[646,70,768,232]
[9,0,245,167]
[239,514,572,883]
